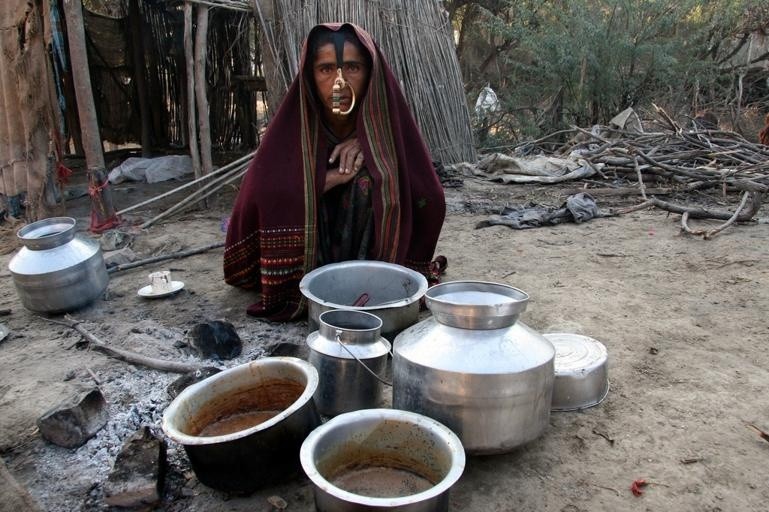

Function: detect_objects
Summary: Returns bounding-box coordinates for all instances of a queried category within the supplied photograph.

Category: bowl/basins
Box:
[543,331,611,411]
[298,258,429,337]
[298,406,467,511]
[162,356,320,492]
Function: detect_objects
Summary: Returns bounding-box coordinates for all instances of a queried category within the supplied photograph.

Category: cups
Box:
[148,270,172,294]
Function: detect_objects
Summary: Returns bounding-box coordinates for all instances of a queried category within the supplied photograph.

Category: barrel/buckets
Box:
[307,310,394,415]
[8,217,110,314]
[393,280,554,456]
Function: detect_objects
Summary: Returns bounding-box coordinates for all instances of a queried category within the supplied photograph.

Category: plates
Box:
[137,281,185,299]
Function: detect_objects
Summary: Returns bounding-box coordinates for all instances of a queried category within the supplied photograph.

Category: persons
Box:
[223,22,445,324]
